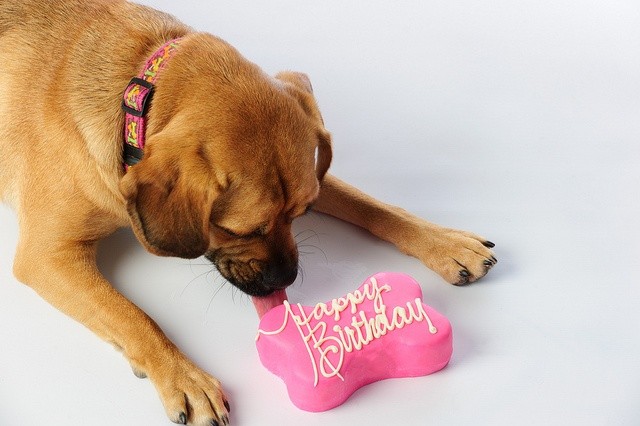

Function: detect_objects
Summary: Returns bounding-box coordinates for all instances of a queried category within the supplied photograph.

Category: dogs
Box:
[1,0,500,426]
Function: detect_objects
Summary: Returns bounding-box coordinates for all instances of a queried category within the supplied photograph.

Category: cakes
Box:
[257,273,453,413]
